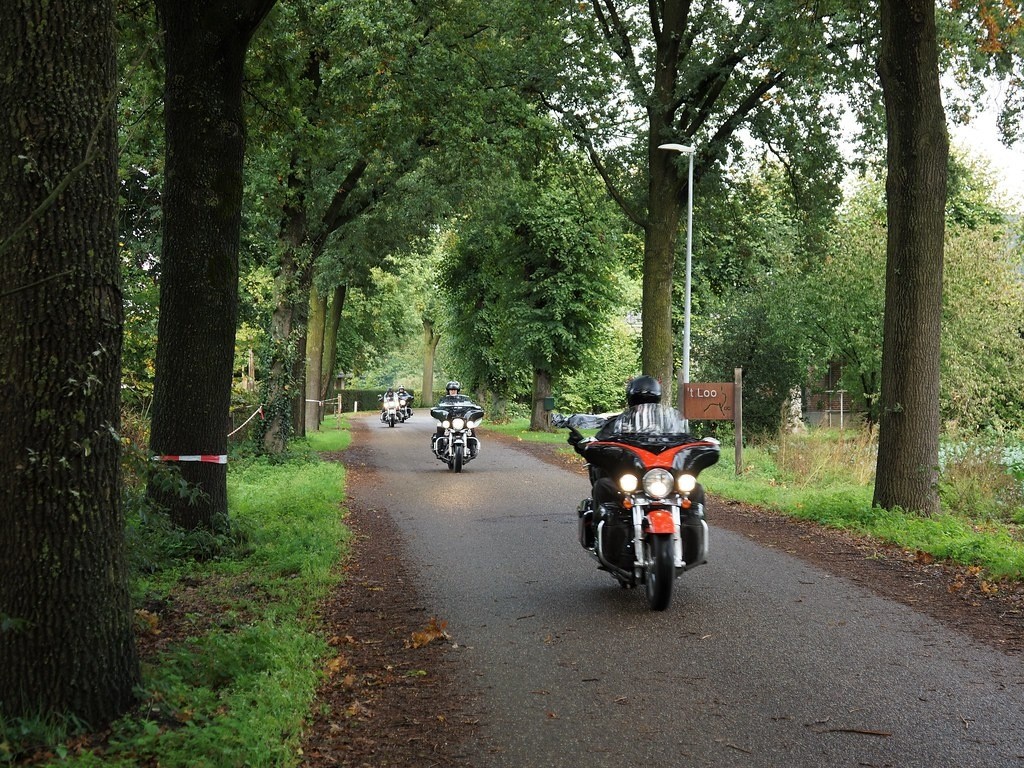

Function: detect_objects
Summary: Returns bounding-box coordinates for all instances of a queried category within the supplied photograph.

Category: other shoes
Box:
[592,536,598,555]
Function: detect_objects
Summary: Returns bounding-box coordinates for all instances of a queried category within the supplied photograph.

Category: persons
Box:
[566,375,720,565]
[430,381,484,459]
[377,385,414,422]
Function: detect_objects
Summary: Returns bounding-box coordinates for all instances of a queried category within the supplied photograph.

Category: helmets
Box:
[387,387,394,392]
[398,385,405,390]
[625,375,662,407]
[446,381,460,392]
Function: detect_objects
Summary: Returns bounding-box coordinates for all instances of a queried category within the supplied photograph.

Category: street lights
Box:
[657,140,695,384]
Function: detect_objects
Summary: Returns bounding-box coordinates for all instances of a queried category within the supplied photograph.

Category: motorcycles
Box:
[429,394,484,474]
[561,402,722,612]
[395,391,414,424]
[377,391,403,428]
